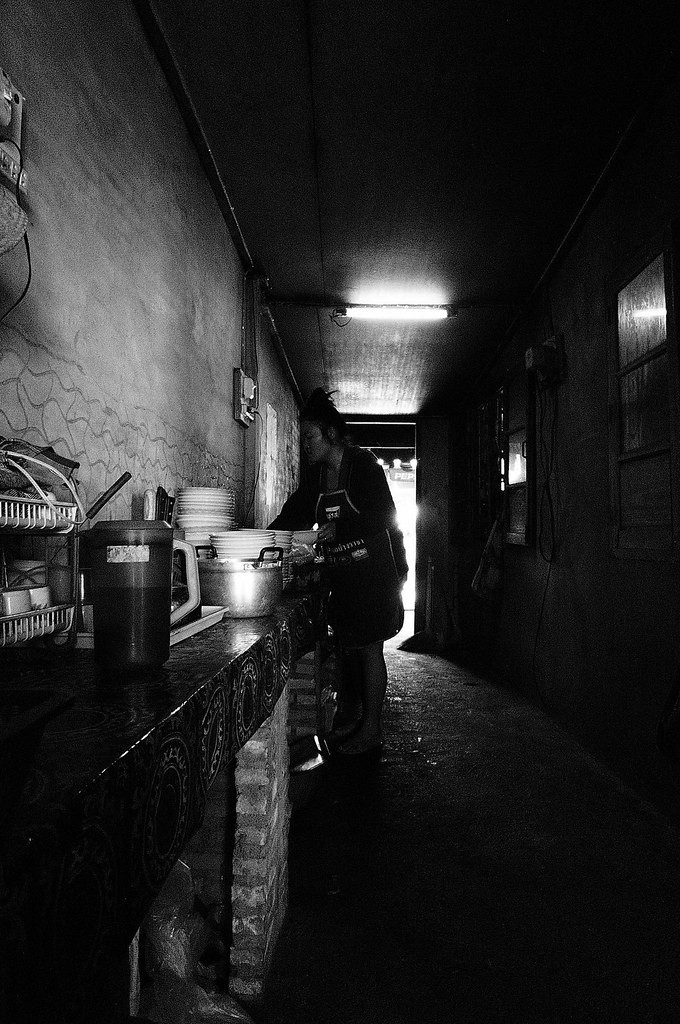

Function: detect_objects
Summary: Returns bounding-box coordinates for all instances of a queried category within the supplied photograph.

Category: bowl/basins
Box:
[295,530,322,546]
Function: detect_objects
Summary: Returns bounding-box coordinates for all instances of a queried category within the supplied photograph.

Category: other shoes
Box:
[324,727,353,742]
[338,730,385,757]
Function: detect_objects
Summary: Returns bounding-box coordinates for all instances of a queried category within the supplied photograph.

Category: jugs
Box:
[83,519,202,672]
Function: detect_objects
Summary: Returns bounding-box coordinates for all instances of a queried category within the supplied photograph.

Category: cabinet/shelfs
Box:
[0,475,80,655]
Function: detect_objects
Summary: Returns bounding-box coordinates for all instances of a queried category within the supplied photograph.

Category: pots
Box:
[194,544,284,618]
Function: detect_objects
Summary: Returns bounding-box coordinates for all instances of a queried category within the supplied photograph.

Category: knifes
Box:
[142,486,175,529]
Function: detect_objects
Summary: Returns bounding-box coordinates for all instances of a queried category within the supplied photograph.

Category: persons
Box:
[268,386,407,753]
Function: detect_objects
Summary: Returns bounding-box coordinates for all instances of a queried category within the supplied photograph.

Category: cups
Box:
[0,583,52,615]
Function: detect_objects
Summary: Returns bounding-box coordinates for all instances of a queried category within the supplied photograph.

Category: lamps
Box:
[336,308,457,318]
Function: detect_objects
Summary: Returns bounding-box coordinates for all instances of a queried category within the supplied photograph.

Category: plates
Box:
[175,486,320,589]
